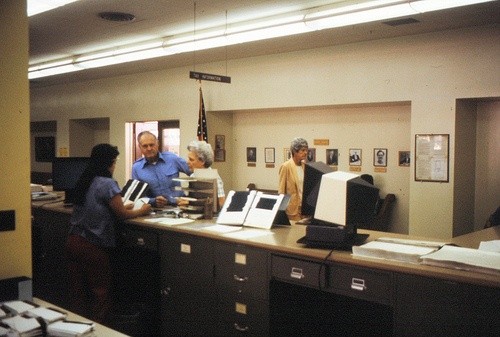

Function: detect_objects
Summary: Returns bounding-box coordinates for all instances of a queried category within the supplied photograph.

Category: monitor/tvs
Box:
[52,156,91,204]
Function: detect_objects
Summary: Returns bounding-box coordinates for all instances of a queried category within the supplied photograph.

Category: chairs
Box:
[371,193,396,230]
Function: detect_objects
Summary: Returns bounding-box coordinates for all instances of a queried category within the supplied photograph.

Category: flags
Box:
[197,97,208,143]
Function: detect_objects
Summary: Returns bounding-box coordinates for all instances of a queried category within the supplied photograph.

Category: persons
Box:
[187,141,225,207]
[278,138,308,221]
[61,143,153,325]
[131,131,192,208]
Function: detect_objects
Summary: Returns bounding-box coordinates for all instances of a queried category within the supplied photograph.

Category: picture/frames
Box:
[307,148,316,160]
[285,148,292,162]
[326,149,338,166]
[349,149,362,166]
[215,135,225,162]
[373,148,388,166]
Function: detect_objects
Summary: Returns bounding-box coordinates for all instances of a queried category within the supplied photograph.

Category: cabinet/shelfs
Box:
[32,193,500,336]
